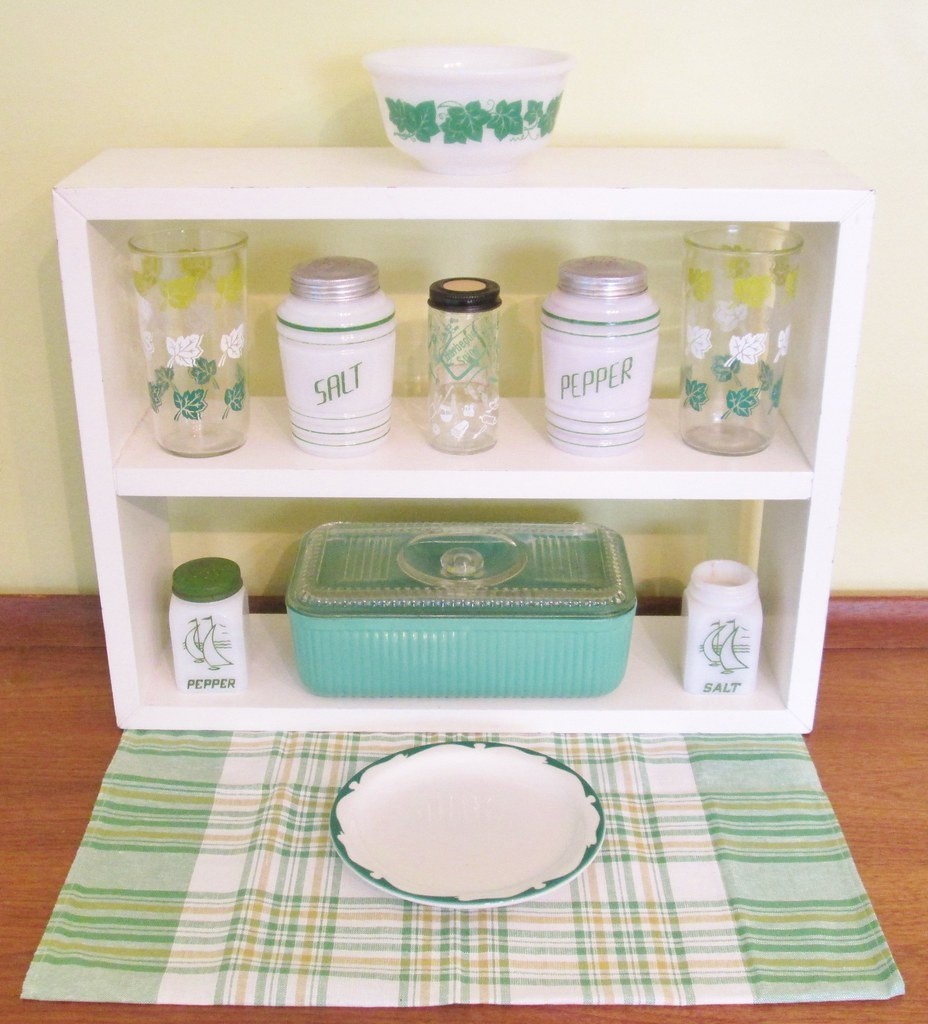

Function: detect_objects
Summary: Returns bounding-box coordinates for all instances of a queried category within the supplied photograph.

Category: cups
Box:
[127,229,248,458]
[680,225,804,456]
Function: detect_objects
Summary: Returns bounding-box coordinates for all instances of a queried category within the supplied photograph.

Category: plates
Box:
[328,740,606,911]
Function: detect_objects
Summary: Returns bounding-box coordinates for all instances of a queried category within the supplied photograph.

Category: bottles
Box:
[539,257,661,457]
[682,559,763,694]
[168,557,249,696]
[427,277,502,454]
[276,257,397,457]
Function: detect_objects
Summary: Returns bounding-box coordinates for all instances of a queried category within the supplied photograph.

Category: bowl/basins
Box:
[285,519,637,700]
[362,48,570,174]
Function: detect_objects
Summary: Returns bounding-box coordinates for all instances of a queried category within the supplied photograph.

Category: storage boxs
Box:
[284,520,637,696]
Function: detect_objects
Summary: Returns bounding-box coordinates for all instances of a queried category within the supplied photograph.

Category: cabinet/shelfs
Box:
[51,141,879,736]
